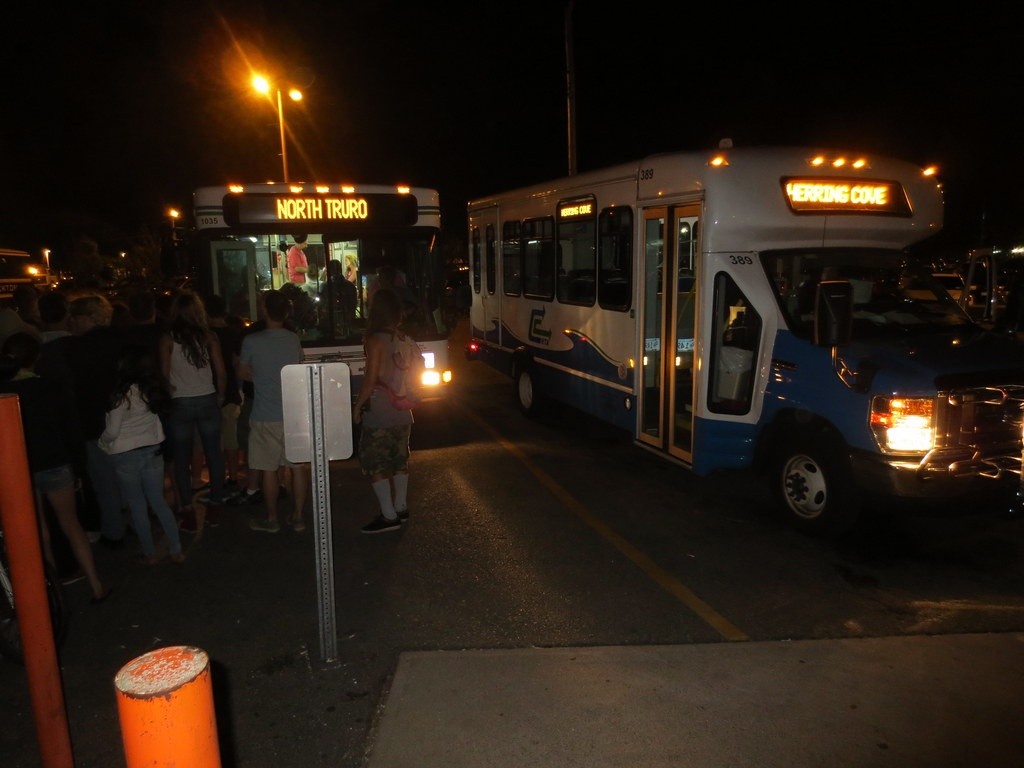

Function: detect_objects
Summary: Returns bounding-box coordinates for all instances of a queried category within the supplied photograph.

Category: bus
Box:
[463,146,1024,541]
[191,182,453,415]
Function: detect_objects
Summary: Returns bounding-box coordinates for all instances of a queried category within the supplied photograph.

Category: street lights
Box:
[41,248,51,270]
[247,72,305,184]
[120,252,127,280]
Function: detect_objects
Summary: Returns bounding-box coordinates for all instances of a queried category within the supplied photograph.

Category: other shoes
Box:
[179,511,198,533]
[285,513,307,531]
[171,552,188,562]
[97,535,124,549]
[360,514,402,534]
[237,486,261,499]
[144,555,162,565]
[204,503,224,528]
[380,508,410,522]
[249,518,280,532]
[91,586,113,603]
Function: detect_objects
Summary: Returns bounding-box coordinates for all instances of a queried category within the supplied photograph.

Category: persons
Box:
[721,254,949,350]
[0,233,420,611]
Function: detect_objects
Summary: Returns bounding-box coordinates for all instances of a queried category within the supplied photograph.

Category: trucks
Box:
[0,249,50,300]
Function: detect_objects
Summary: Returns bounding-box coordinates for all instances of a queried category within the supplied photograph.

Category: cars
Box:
[445,255,480,292]
[901,274,974,308]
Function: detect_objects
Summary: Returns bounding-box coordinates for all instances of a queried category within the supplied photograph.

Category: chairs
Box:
[498,267,922,326]
